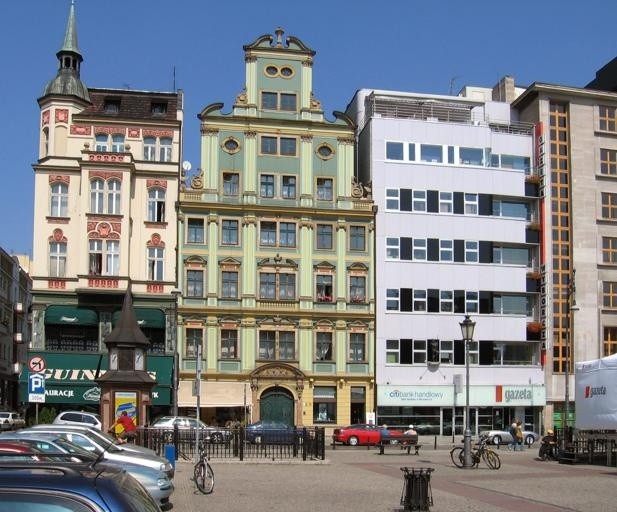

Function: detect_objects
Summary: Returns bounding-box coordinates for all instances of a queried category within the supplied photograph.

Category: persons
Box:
[377,424,389,444]
[403,423,418,454]
[507,418,519,450]
[511,419,526,452]
[106,410,136,442]
[537,429,556,460]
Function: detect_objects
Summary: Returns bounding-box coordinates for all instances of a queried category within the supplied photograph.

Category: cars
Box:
[0,410,175,512]
[245,420,304,445]
[152,416,234,444]
[332,424,402,445]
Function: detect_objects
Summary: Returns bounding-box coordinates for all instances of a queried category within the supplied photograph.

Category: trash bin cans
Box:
[400,467,435,512]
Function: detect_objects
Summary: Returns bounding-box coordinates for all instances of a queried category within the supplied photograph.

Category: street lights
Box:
[459,314,476,470]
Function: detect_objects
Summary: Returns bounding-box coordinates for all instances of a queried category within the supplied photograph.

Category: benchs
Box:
[374,434,423,455]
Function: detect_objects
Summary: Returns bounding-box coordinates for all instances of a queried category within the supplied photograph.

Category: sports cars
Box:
[481,427,539,445]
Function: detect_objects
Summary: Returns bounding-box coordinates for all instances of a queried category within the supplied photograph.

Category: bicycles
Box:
[450,439,501,470]
[194,442,214,494]
